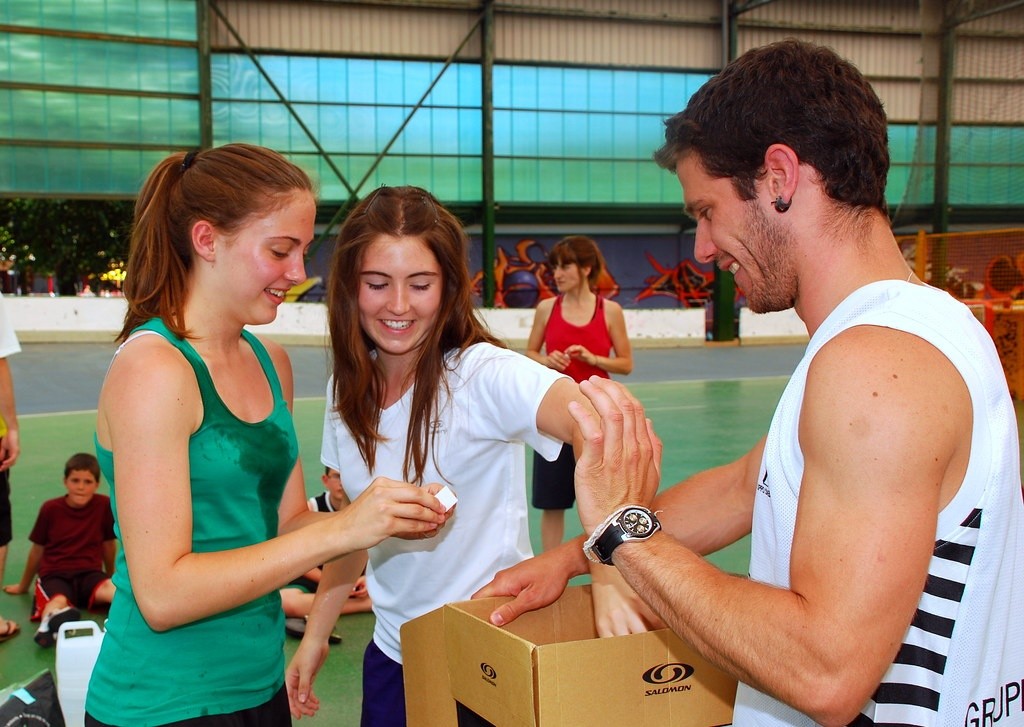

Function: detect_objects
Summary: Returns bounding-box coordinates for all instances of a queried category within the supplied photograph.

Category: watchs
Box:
[580,504,665,566]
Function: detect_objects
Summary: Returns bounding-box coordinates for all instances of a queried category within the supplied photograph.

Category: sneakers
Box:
[34,606,80,649]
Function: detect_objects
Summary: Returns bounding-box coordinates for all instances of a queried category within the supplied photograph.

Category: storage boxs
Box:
[397,582,738,727]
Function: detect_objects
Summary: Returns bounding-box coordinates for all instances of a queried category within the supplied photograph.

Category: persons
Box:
[1,452,117,649]
[284,184,671,726]
[82,139,460,727]
[469,34,1024,727]
[279,461,372,617]
[524,234,633,554]
[0,287,22,643]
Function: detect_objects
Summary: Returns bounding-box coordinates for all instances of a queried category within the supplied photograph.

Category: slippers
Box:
[0,619,18,641]
